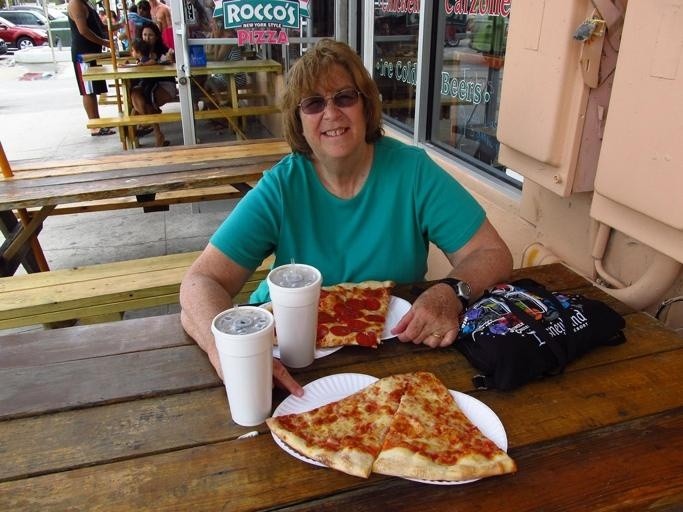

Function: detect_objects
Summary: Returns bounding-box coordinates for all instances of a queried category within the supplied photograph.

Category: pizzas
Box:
[265,281,393,348]
[265,372,518,480]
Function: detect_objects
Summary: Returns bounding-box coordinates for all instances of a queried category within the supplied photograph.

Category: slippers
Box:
[135,125,154,137]
[91,127,116,136]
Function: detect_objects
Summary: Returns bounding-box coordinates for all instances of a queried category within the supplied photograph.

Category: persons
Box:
[179,39,514,397]
[67,0,247,147]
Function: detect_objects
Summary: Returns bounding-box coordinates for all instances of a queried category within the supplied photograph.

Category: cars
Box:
[0,5,72,54]
[467,2,513,70]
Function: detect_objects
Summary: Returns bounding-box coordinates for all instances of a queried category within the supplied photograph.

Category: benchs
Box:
[1,247,275,333]
[14,178,260,217]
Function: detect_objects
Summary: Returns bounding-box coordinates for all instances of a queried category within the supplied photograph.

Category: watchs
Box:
[438,277,471,313]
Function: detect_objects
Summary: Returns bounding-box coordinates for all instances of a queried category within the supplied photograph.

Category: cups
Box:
[209,306,275,428]
[267,263,324,369]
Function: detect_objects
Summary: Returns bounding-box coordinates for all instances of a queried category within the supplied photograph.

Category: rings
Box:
[431,332,443,340]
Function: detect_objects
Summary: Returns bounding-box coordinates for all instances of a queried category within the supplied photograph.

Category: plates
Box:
[268,372,510,487]
[251,288,419,364]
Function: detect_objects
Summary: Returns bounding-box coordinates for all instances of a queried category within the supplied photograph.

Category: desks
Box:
[83,54,282,150]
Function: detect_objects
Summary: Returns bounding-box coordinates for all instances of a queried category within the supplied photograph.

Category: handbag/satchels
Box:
[461,279,626,392]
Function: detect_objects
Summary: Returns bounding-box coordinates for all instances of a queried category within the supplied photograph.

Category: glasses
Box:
[297,89,362,114]
